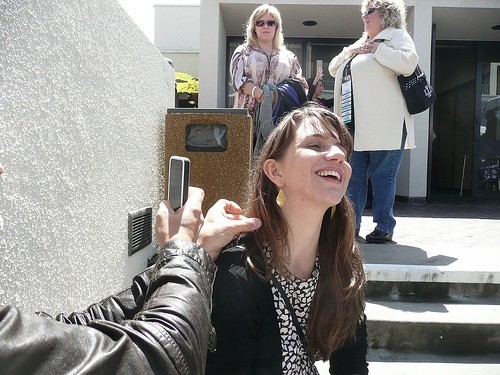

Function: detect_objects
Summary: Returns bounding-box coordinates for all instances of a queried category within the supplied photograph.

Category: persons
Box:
[230,3,309,153]
[329,0,419,244]
[205,101,370,375]
[306,67,334,109]
[0,165,262,375]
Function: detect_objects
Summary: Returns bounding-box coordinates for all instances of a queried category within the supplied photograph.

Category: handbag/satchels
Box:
[398,63,437,115]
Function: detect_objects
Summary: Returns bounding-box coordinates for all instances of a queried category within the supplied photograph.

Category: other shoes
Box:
[365,229,392,244]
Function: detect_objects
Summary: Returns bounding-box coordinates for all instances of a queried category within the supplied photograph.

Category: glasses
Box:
[365,8,378,15]
[255,20,275,27]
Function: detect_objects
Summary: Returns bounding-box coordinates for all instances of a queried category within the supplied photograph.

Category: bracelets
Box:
[260,92,264,99]
[252,86,258,97]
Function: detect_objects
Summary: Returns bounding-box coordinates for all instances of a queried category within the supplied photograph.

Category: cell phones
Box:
[167,156,190,213]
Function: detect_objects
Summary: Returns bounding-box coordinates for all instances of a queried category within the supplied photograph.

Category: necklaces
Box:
[257,45,273,67]
